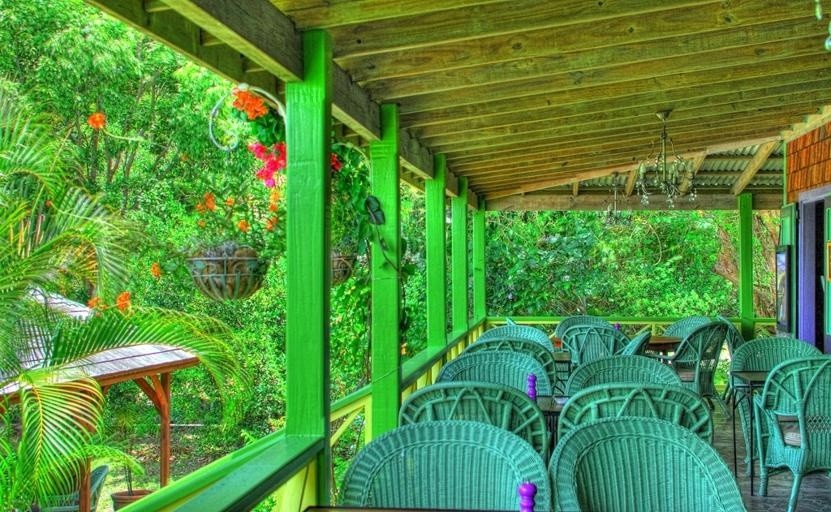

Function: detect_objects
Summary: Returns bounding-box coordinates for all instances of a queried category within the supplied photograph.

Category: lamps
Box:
[604,171,632,227]
[636,110,698,210]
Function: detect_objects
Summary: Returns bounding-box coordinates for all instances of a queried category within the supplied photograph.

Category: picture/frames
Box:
[776,242,791,331]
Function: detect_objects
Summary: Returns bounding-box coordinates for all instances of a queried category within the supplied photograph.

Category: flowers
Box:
[328,143,416,333]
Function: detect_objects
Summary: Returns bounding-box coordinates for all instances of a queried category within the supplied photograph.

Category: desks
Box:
[0,286,199,489]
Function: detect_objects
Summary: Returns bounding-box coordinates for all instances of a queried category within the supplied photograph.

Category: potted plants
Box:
[104,384,154,512]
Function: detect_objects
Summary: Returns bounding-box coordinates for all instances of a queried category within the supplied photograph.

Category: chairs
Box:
[39,465,109,512]
